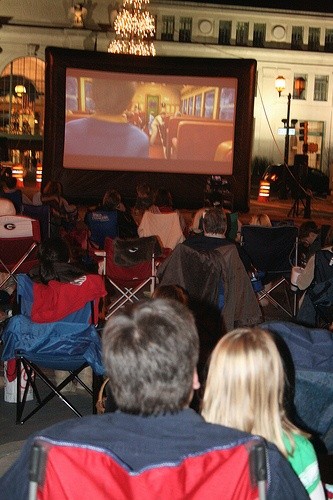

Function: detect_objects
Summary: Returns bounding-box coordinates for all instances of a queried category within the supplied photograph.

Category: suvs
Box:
[263,165,329,198]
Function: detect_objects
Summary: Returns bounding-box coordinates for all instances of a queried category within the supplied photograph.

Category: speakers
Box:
[295,155,309,177]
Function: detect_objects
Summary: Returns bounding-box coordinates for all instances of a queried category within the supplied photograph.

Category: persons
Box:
[65,78,182,159]
[0,168,333,500]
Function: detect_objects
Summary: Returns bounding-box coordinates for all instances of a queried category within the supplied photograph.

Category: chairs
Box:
[84,210,119,249]
[136,211,186,249]
[0,437,270,500]
[0,273,109,426]
[20,204,55,247]
[188,210,240,240]
[0,215,50,303]
[257,317,333,459]
[158,244,263,330]
[241,225,299,319]
[88,236,172,320]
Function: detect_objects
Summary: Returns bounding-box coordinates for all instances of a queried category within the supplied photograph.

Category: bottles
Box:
[246,262,257,281]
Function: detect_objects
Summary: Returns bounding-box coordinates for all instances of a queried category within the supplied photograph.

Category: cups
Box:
[290,266,304,291]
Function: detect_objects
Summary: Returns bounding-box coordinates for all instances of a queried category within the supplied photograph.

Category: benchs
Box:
[160,115,233,160]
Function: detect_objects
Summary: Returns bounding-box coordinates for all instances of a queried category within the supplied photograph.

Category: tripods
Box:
[287,178,305,218]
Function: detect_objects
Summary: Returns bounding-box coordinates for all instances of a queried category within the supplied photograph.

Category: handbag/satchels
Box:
[4,360,34,402]
